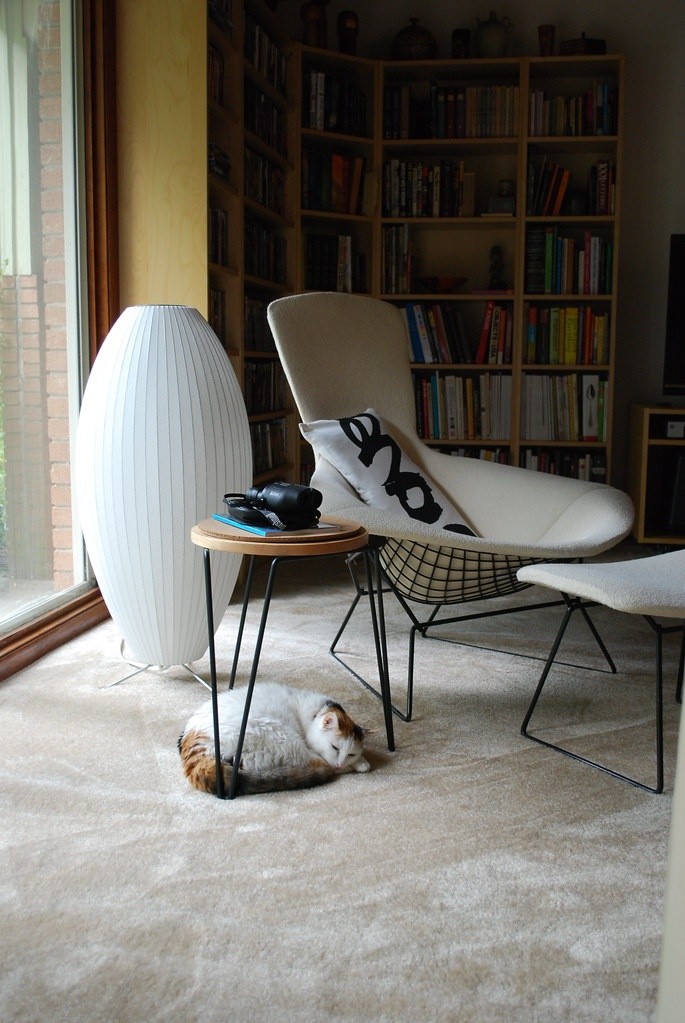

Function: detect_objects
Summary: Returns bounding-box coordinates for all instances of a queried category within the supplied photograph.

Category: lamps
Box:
[70,304,253,694]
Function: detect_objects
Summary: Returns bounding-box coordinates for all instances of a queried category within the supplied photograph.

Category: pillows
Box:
[297,406,481,536]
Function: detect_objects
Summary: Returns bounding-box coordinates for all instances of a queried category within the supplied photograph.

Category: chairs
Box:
[514,550,685,794]
[265,291,636,724]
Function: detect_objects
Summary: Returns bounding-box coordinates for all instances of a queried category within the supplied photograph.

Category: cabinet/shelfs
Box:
[627,401,685,553]
[113,0,626,589]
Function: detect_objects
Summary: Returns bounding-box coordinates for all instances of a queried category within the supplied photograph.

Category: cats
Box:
[177,683,374,797]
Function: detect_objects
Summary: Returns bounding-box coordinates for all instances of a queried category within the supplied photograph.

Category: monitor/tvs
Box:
[661,234,685,396]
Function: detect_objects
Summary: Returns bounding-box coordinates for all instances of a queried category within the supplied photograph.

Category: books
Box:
[207,0,621,488]
